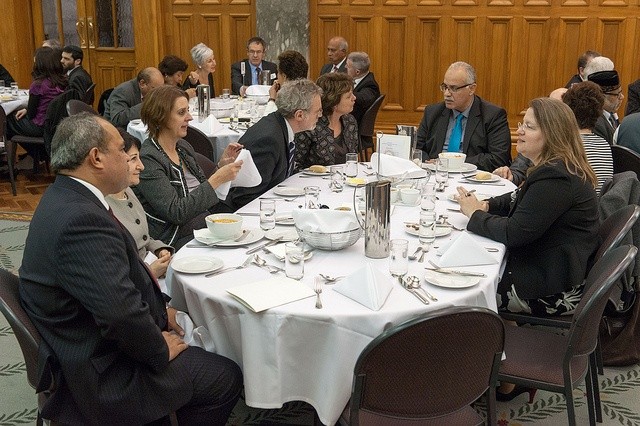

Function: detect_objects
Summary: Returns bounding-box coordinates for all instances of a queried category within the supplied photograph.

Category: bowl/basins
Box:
[206,212,242,240]
[438,152,467,169]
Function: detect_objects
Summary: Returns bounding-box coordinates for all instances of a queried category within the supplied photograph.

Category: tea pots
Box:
[197,84,214,123]
[354,181,392,257]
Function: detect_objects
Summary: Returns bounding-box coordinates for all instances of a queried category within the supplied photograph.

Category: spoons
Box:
[409,246,422,260]
[407,275,436,302]
[320,273,346,282]
[362,162,372,168]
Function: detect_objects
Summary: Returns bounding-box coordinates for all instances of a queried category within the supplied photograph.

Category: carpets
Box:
[0,219,640,425]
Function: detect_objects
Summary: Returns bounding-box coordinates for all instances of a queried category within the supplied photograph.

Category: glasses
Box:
[604,89,622,96]
[440,81,476,90]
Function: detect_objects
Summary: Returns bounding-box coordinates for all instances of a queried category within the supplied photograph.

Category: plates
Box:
[265,226,299,242]
[274,186,307,196]
[306,166,331,176]
[407,222,453,236]
[172,255,227,273]
[194,225,266,245]
[437,162,478,173]
[425,267,481,285]
[467,177,499,182]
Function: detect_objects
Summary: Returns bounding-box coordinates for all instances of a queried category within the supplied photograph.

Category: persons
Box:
[456,98,602,402]
[225,78,322,208]
[1,46,69,178]
[18,113,243,424]
[138,86,243,250]
[103,67,164,128]
[320,36,348,74]
[562,81,614,195]
[60,44,94,104]
[293,71,360,169]
[231,36,278,96]
[107,126,176,293]
[586,70,620,144]
[492,87,568,184]
[183,41,215,98]
[564,52,614,88]
[43,38,65,50]
[411,61,512,170]
[157,53,200,97]
[344,51,380,131]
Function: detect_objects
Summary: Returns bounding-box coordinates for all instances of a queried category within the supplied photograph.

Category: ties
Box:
[335,66,339,73]
[109,207,122,229]
[449,114,464,152]
[610,115,618,130]
[256,67,263,84]
[289,140,296,178]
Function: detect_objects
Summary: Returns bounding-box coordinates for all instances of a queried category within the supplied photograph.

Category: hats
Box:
[588,69,621,92]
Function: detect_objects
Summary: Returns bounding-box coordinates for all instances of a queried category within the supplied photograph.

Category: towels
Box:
[370,153,426,179]
[197,114,222,133]
[332,265,393,310]
[143,251,168,294]
[161,312,215,353]
[430,230,498,268]
[214,150,262,201]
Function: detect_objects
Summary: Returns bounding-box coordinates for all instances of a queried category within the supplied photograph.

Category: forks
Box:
[206,254,254,276]
[259,195,299,202]
[419,248,428,263]
[256,255,284,273]
[315,288,324,308]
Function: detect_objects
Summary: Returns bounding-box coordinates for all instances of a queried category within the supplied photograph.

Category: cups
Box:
[399,125,416,159]
[0,79,7,97]
[390,187,400,202]
[347,154,358,178]
[12,83,19,101]
[220,88,230,105]
[412,149,422,170]
[305,186,321,209]
[422,182,436,212]
[330,165,344,193]
[391,240,408,276]
[400,189,420,206]
[260,199,275,231]
[229,110,239,131]
[420,213,438,243]
[284,242,305,280]
[437,159,449,184]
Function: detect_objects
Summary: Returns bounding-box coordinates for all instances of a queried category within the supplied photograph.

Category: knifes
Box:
[245,236,284,255]
[228,125,240,136]
[398,277,429,304]
[186,244,248,249]
[459,181,505,186]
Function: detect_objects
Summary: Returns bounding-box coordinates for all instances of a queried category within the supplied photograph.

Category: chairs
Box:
[85,82,98,107]
[1,269,59,426]
[185,125,216,160]
[342,307,504,425]
[97,87,114,116]
[503,201,640,422]
[357,94,386,162]
[6,90,84,196]
[495,244,638,426]
[610,144,640,173]
[66,99,95,115]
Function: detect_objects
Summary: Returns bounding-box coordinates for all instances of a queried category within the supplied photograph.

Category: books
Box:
[227,277,316,311]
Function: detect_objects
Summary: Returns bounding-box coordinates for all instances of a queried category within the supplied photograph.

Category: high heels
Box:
[496,378,537,403]
